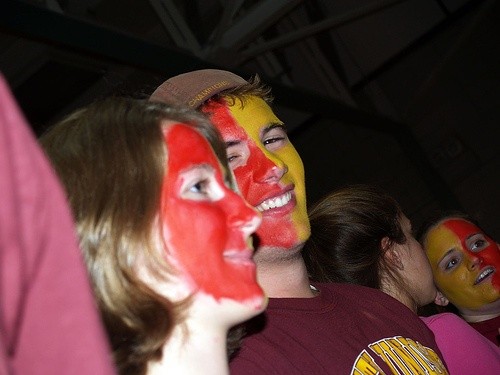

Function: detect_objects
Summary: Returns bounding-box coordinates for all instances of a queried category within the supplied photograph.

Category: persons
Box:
[417,210,500,349]
[38,99,269,375]
[300,190,500,375]
[148,69,451,375]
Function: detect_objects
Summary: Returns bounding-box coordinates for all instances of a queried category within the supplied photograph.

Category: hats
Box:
[149,69,250,110]
